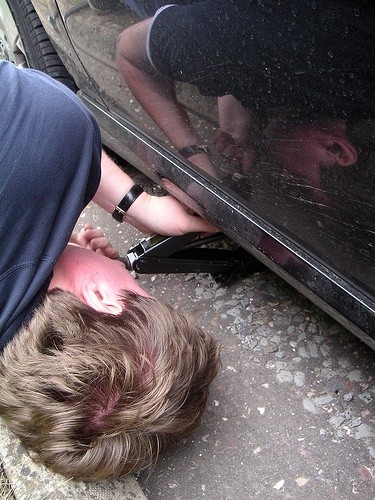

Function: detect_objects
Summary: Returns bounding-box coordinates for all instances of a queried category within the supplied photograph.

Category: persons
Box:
[85,0,374,234]
[0,58,227,488]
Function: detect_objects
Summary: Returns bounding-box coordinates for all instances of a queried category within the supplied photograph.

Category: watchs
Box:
[176,143,213,159]
[112,183,145,223]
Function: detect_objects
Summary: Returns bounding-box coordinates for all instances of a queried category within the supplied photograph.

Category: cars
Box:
[0,1,374,351]
